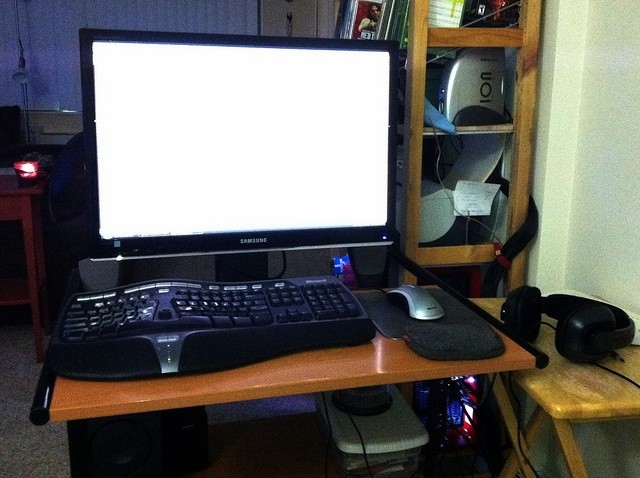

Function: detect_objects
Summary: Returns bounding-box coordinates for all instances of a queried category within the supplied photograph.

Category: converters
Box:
[412,373,487,478]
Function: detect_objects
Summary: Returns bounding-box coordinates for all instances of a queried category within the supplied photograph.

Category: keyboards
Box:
[45,275,377,381]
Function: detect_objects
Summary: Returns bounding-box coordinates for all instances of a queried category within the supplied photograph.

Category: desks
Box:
[468,298,640,477]
[0,167,50,358]
[50,285,536,478]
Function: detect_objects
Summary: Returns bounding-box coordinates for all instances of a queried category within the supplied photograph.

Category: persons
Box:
[358,4,381,32]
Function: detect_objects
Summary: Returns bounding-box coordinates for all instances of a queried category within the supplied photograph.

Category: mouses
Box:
[384,283,447,323]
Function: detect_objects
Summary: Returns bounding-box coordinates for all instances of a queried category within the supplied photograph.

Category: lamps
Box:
[12,37,31,145]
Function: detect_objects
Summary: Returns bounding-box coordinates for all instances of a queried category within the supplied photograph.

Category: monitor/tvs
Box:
[79,27,397,281]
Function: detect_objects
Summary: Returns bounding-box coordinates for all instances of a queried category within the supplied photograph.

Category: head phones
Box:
[500,287,636,365]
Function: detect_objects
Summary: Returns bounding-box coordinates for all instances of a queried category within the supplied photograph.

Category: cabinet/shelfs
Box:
[332,0,544,450]
